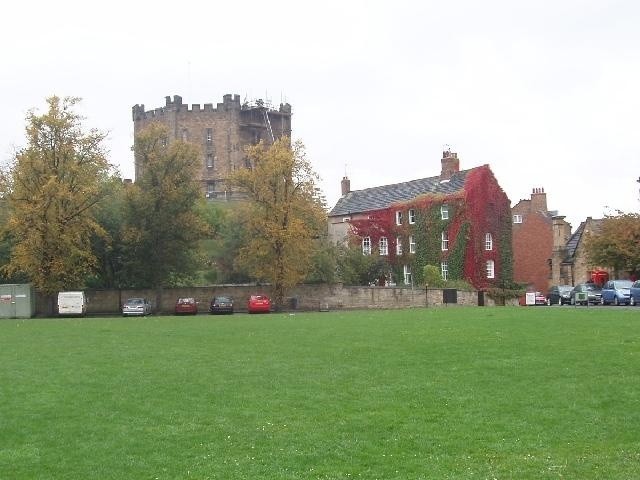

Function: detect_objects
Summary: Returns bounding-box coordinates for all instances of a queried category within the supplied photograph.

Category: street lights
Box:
[426,282,429,301]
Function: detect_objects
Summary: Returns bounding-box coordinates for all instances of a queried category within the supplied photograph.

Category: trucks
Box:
[56,290,88,318]
[0,284,38,319]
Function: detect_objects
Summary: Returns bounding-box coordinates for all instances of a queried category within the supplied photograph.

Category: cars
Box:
[569,283,602,305]
[209,296,235,315]
[599,278,636,305]
[629,279,640,306]
[121,296,152,317]
[246,294,273,313]
[174,296,200,315]
[535,291,547,305]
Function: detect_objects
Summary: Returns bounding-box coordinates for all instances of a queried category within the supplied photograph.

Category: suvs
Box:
[546,285,575,306]
